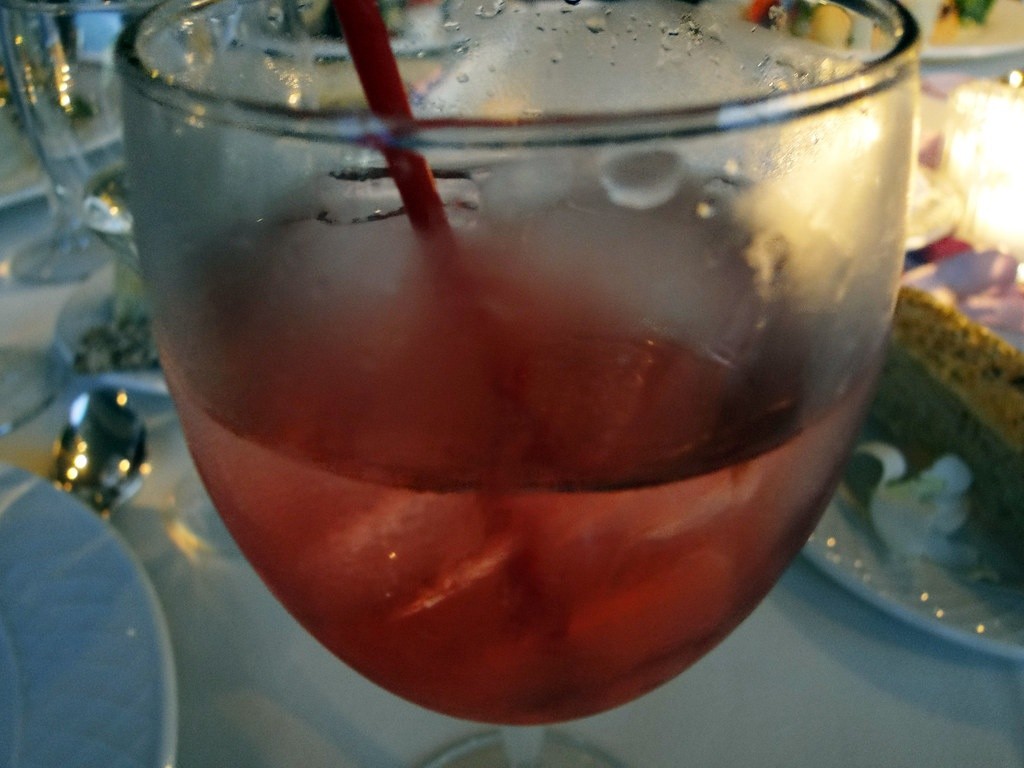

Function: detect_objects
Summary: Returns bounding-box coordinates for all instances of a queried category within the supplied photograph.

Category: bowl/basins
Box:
[702,0,1024,93]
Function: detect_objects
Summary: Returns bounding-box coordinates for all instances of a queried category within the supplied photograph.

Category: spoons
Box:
[49,385,153,518]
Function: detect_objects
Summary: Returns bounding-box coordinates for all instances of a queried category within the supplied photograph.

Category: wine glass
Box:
[0,0,142,285]
[115,0,921,768]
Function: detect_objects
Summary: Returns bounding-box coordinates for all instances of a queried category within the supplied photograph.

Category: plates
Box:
[799,249,1024,661]
[0,464,178,768]
